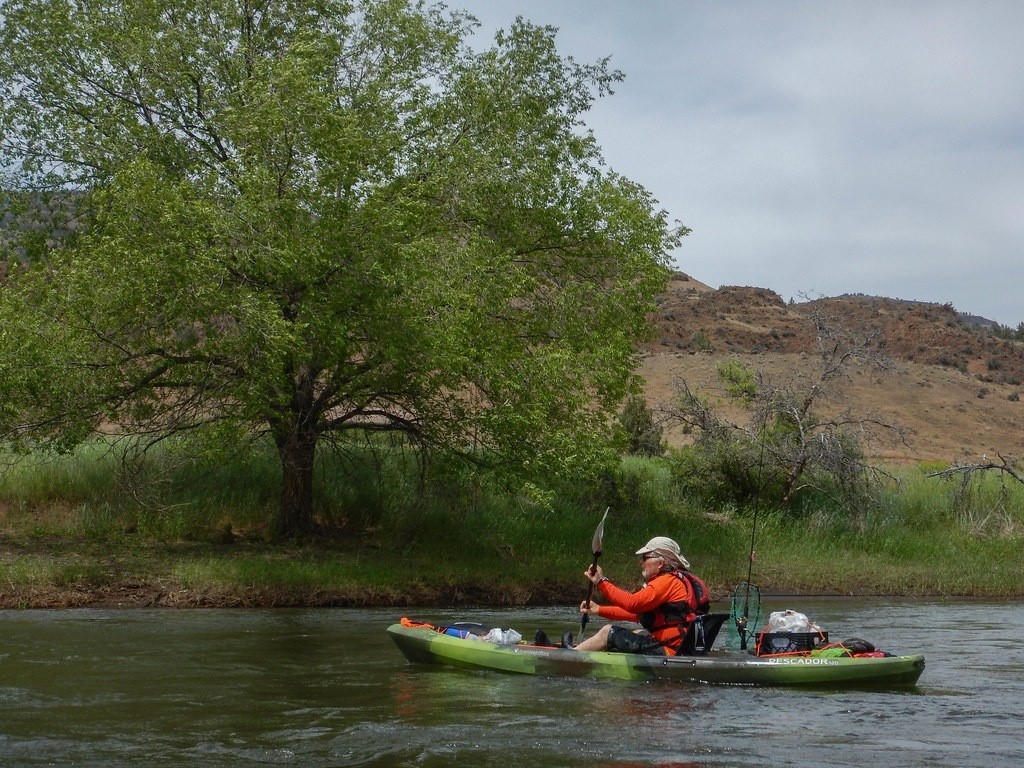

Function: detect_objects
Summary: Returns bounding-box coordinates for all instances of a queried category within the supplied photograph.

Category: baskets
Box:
[755,632,828,658]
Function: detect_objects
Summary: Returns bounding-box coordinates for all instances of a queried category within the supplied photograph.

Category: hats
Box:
[634,537,690,568]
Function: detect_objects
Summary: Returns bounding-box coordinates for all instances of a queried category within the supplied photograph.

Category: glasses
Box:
[642,555,658,561]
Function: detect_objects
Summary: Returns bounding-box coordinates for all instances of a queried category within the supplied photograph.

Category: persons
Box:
[534,536,698,657]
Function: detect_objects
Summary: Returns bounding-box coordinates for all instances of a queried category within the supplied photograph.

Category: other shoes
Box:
[562,631,574,648]
[535,630,550,646]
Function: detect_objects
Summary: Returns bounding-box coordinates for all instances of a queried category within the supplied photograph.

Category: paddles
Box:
[572,503,612,644]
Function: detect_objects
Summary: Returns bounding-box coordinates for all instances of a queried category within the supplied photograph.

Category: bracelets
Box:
[596,576,609,588]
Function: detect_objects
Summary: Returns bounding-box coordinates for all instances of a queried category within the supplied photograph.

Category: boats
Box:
[386,615,927,692]
